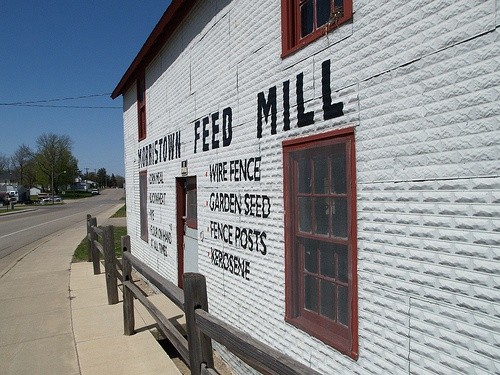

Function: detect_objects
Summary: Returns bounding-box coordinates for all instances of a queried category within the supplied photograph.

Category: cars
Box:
[37,193,64,202]
[92,189,99,195]
